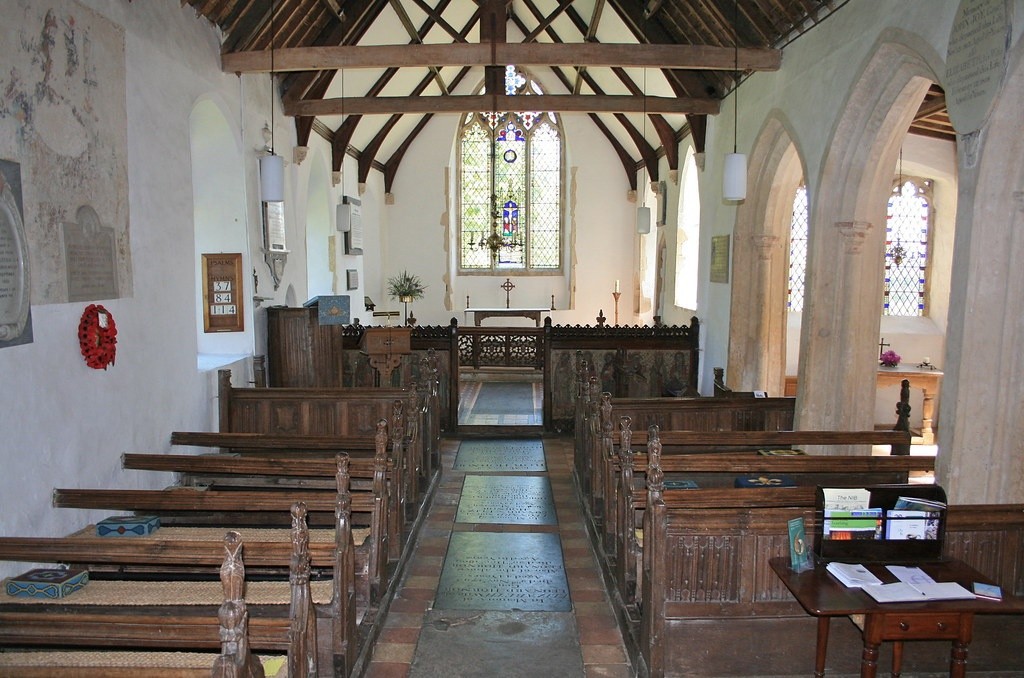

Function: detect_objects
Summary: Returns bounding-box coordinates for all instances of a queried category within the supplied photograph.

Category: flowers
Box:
[877,351,901,367]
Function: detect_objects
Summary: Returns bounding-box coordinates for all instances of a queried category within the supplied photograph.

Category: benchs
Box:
[573,351,1023,678]
[0,347,441,678]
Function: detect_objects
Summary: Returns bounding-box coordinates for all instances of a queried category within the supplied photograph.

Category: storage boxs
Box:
[95,515,160,537]
[6,568,89,600]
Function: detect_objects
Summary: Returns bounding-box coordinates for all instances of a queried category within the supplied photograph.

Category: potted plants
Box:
[386,271,426,303]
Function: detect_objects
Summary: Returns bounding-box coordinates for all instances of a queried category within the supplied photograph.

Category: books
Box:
[972,582,1002,602]
[826,562,976,604]
[822,488,946,541]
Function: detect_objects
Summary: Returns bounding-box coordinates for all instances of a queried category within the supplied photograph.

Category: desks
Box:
[768,556,1024,677]
[464,308,550,370]
[877,361,944,445]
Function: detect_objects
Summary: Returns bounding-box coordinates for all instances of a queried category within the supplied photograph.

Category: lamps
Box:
[637,0,650,235]
[336,0,350,232]
[723,0,746,201]
[261,0,285,203]
[467,13,525,263]
[889,144,907,266]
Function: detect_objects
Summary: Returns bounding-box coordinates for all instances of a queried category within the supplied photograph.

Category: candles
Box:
[923,357,930,364]
[615,280,619,293]
